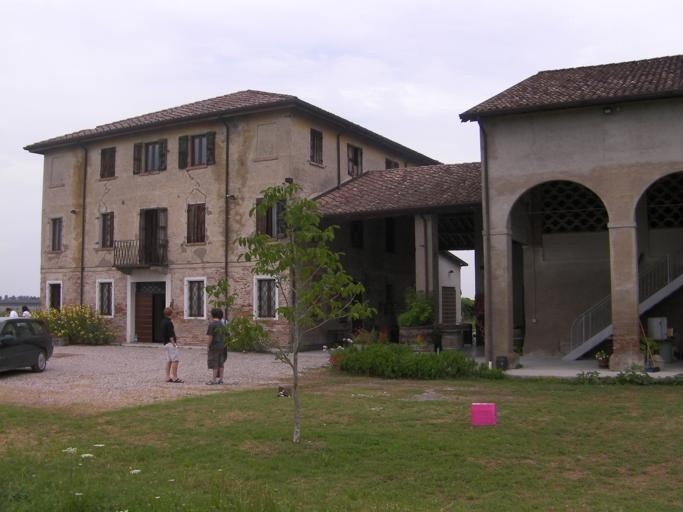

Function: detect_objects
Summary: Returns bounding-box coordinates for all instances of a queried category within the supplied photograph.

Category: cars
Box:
[0,317,53,374]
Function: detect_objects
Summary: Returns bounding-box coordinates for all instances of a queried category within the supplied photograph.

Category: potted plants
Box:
[595,350,609,368]
[396,286,435,352]
[639,337,664,371]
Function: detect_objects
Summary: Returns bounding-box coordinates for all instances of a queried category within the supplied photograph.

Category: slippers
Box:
[167,377,185,383]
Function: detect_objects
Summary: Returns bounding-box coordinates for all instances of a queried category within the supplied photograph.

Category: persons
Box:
[21,306,32,316]
[5,307,18,317]
[161,306,185,383]
[205,307,227,385]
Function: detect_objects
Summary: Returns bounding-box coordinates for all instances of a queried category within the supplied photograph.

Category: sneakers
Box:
[205,379,224,385]
